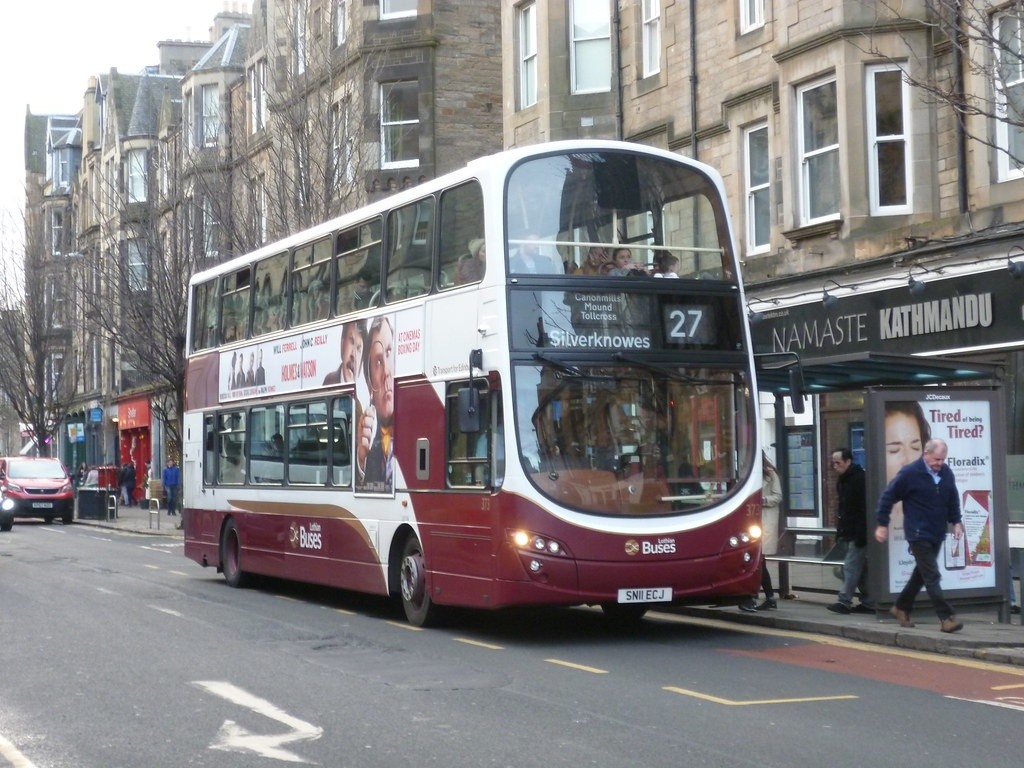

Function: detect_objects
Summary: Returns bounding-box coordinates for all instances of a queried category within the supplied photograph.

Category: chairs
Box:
[293,415,352,467]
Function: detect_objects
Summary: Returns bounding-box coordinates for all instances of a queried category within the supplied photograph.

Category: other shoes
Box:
[754,599,777,612]
[892,607,915,629]
[742,603,758,612]
[828,603,849,614]
[849,604,877,615]
[941,616,963,631]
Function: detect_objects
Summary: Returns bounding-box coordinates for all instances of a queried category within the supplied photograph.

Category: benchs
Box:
[764,527,847,565]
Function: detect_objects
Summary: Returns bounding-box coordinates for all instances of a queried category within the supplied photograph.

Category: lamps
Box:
[747,297,779,325]
[1007,245,1024,279]
[908,264,944,298]
[823,280,858,309]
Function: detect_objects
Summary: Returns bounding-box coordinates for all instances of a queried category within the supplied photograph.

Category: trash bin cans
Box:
[77,489,118,520]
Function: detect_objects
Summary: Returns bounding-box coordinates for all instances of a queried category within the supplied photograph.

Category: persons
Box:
[743,449,782,611]
[77,462,98,486]
[884,401,932,529]
[144,460,151,470]
[874,439,963,632]
[475,424,539,486]
[635,403,705,509]
[462,238,486,282]
[227,348,265,389]
[509,230,680,278]
[269,433,283,457]
[118,461,138,507]
[826,447,876,614]
[347,271,380,312]
[321,315,394,494]
[162,459,181,516]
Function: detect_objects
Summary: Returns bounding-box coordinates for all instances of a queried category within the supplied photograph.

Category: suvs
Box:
[0,456,76,532]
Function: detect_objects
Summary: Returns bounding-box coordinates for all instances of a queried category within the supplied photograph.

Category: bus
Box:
[179,136,808,630]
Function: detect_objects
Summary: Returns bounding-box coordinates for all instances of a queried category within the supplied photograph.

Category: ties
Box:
[385,437,394,491]
